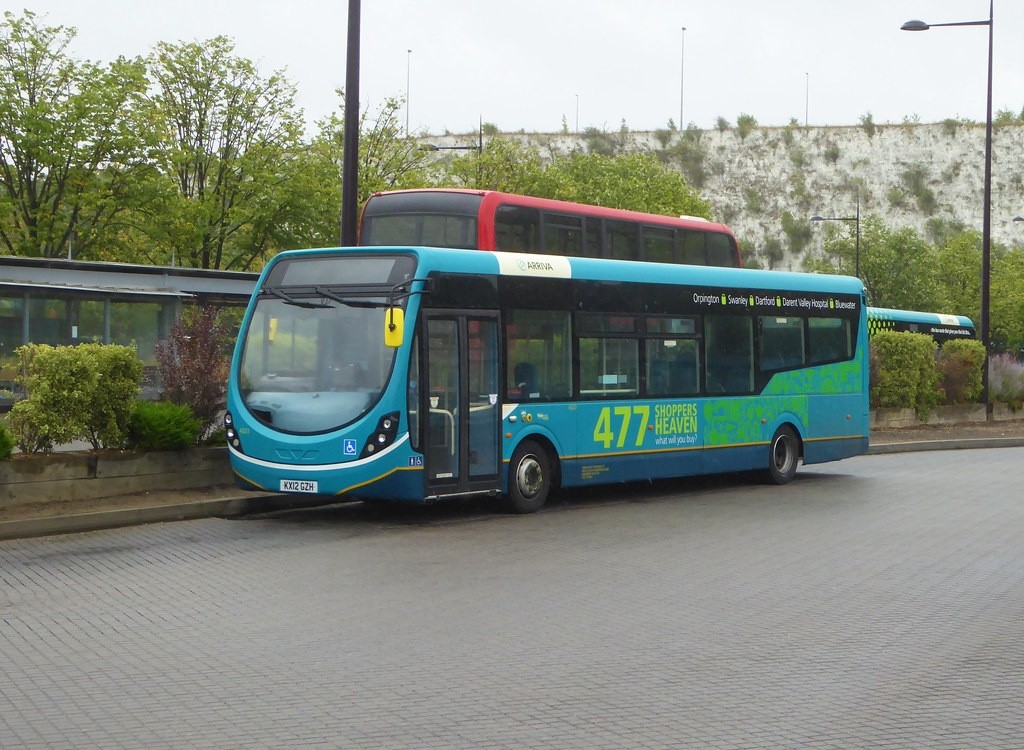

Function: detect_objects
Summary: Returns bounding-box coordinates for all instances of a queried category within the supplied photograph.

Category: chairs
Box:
[640,329,848,397]
[514,363,539,403]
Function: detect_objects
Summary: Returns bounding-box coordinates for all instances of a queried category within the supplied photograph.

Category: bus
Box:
[356,188,741,270]
[223,246,870,514]
[866,307,978,339]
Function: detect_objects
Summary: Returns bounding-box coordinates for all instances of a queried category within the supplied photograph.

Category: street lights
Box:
[902,18,993,420]
